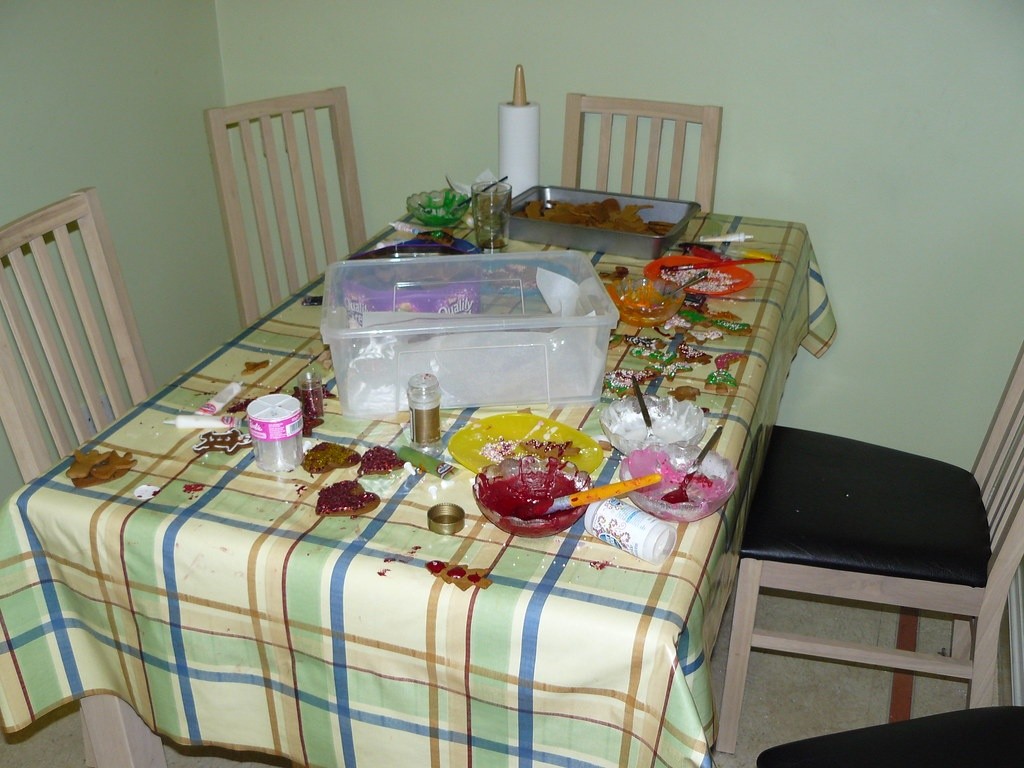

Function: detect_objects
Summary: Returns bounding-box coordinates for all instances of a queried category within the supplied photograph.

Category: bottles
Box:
[297,370,325,419]
[406,374,444,458]
[247,393,303,473]
[583,496,678,566]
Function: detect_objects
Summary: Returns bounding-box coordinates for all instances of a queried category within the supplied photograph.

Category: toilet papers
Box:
[498,101,539,199]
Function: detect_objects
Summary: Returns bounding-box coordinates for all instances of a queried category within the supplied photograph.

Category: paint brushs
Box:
[645,270,708,314]
[449,176,508,214]
[632,374,652,428]
[660,427,724,506]
[512,474,662,521]
[660,258,764,271]
[677,242,782,262]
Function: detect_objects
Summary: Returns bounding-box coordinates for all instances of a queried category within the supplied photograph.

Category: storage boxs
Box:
[320,248,620,421]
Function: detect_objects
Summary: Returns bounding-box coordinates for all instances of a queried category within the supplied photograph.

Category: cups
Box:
[470,182,512,250]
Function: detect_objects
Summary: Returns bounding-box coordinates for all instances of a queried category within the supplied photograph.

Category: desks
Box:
[0,209,836,768]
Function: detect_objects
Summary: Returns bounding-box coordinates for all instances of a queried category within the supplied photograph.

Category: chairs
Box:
[561,92,723,212]
[203,86,369,329]
[0,186,159,767]
[715,344,1024,753]
[757,706,1024,768]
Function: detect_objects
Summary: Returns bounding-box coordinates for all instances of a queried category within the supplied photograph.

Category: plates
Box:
[643,255,756,297]
[448,412,604,474]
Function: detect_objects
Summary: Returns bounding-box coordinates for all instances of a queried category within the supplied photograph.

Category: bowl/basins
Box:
[619,439,738,522]
[599,394,707,456]
[406,188,472,228]
[471,455,594,539]
[604,273,687,326]
